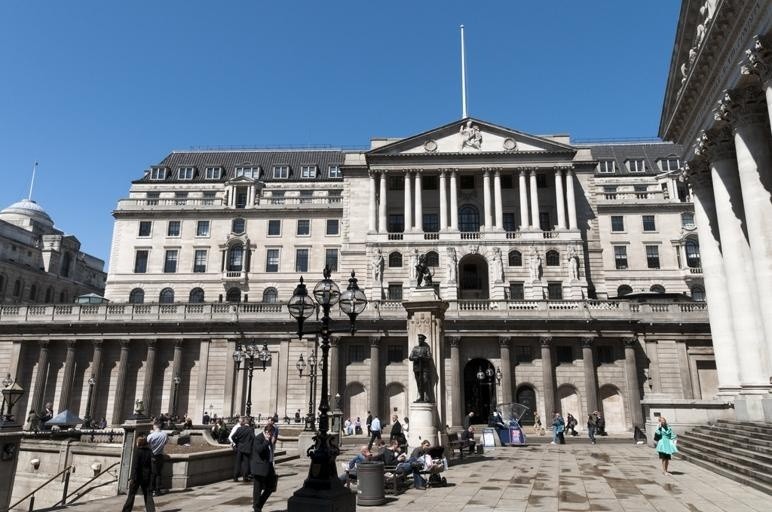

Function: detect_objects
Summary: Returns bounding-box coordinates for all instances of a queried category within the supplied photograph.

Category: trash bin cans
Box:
[355,461,386,506]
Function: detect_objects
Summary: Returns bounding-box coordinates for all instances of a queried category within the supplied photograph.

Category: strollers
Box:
[418,447,448,488]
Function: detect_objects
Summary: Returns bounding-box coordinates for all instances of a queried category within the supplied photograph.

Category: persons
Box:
[39,401,53,432]
[82,409,300,512]
[446,411,505,455]
[533,412,542,434]
[409,334,434,403]
[655,418,678,476]
[372,246,580,281]
[338,411,431,490]
[460,121,480,150]
[27,409,40,432]
[550,411,608,444]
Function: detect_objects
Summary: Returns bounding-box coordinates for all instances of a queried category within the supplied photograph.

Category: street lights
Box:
[0,373,12,418]
[474,364,505,427]
[172,373,181,416]
[83,373,95,426]
[296,346,325,430]
[282,266,370,511]
[0,377,23,429]
[233,336,271,419]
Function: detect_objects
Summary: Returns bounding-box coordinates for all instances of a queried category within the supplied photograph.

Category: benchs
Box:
[447,432,472,457]
[381,454,413,496]
[347,453,378,489]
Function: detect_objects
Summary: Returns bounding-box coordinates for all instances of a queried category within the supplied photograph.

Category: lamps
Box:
[2,377,25,421]
